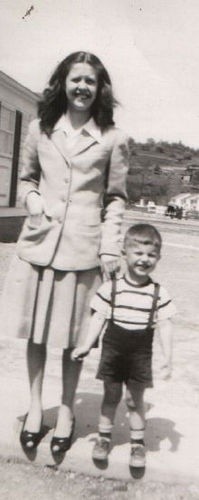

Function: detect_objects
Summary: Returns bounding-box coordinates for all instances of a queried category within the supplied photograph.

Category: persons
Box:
[70,224,177,468]
[0,51,130,454]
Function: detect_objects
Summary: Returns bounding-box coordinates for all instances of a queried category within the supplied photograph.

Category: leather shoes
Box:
[20,412,43,448]
[51,416,75,453]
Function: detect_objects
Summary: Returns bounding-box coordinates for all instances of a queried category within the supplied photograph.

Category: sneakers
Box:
[92,437,111,460]
[129,446,145,467]
